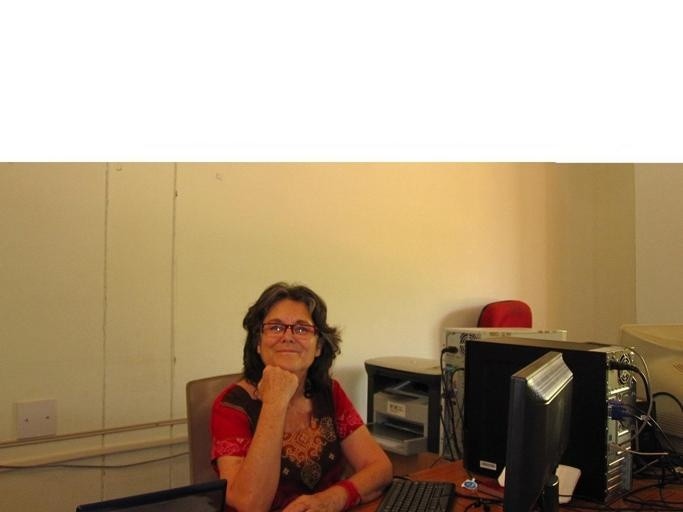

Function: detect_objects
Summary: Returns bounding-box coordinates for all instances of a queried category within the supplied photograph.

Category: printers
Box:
[363,355,444,457]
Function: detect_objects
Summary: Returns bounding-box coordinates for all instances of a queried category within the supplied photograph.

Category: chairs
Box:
[184,373,248,482]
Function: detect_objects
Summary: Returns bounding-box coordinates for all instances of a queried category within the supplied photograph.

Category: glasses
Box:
[259,322,317,336]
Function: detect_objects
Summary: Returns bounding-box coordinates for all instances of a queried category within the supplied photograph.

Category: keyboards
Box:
[375,478,455,512]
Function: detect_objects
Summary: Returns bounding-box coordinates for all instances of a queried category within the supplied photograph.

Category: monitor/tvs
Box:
[504,351,575,511]
[616,324,683,453]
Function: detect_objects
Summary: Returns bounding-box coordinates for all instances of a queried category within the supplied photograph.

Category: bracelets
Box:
[332,478,363,511]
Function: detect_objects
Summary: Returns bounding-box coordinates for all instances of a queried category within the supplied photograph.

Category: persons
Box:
[205,282,396,510]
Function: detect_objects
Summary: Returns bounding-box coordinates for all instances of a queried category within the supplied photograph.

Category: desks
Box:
[80,451,683,510]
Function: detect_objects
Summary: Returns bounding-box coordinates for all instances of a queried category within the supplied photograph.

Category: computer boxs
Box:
[463,337,636,503]
[443,327,567,461]
